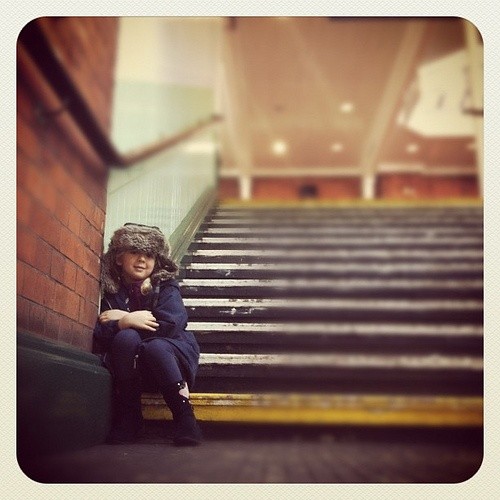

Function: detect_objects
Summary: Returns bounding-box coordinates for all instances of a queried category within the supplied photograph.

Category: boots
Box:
[107,380,147,444]
[160,376,203,446]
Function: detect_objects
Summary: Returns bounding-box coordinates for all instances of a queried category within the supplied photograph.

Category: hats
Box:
[101,222,180,295]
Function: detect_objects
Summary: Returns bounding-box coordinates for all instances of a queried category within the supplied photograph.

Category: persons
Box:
[91,220,202,448]
